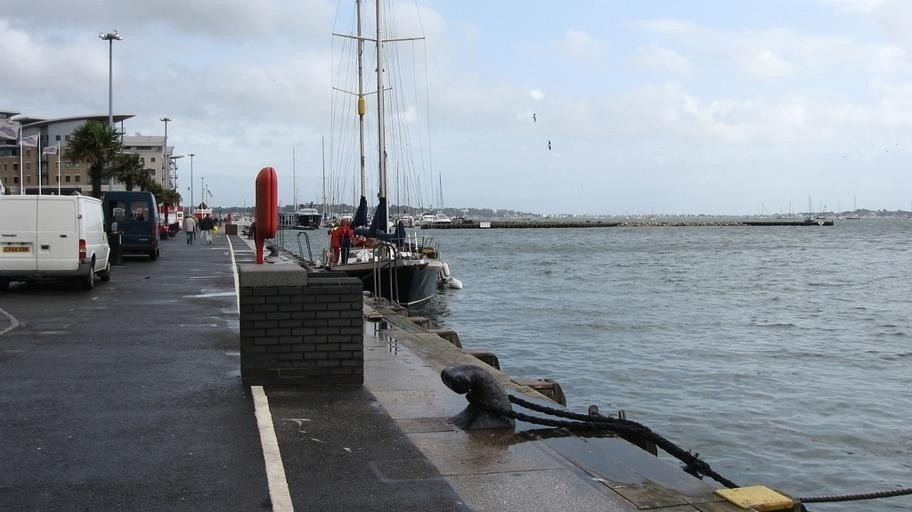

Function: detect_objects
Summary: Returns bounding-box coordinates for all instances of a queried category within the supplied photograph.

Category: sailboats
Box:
[288,134,474,229]
[743,195,865,227]
[315,1,455,312]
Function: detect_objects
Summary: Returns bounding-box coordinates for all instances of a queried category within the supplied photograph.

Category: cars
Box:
[159,218,171,240]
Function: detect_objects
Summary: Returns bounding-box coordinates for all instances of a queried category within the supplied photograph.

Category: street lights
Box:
[188,154,195,213]
[201,176,213,219]
[160,117,171,225]
[100,29,124,190]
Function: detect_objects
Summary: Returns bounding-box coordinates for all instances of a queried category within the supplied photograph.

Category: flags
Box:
[1,121,19,139]
[18,134,39,147]
[42,143,57,155]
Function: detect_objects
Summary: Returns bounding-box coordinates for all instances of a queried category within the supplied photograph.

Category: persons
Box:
[183,213,218,246]
[329,216,356,264]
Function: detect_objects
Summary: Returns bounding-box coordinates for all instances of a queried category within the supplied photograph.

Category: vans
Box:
[100,191,162,260]
[0,192,115,292]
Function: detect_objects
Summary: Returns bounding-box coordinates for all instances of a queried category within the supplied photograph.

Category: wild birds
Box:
[532,112,537,123]
[546,140,552,150]
